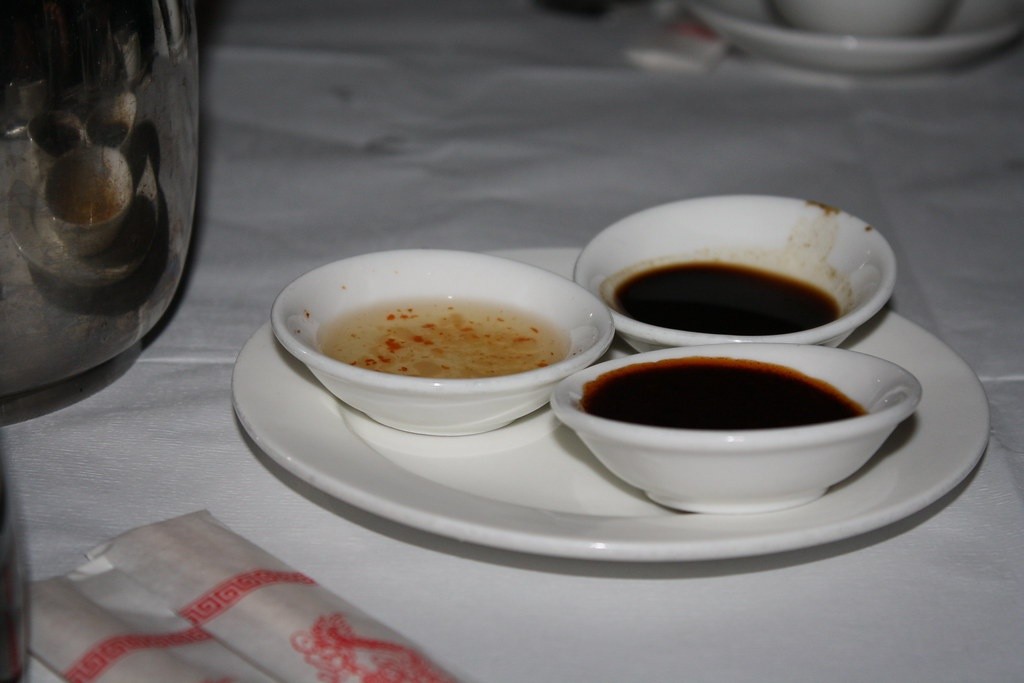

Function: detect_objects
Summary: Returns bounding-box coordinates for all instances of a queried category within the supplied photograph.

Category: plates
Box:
[232,245,991,562]
[690,0,1024,77]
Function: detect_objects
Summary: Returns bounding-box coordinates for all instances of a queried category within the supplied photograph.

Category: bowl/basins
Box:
[572,194,897,354]
[773,0,959,36]
[270,248,615,437]
[550,343,923,514]
